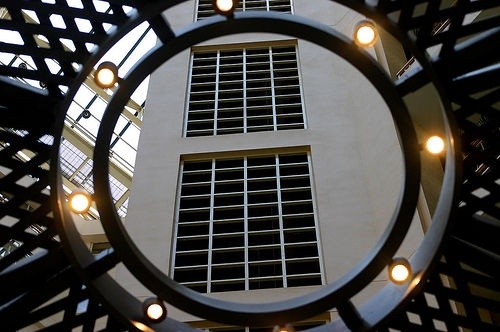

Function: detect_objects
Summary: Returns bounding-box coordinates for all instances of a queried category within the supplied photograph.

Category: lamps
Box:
[67,0,445,324]
